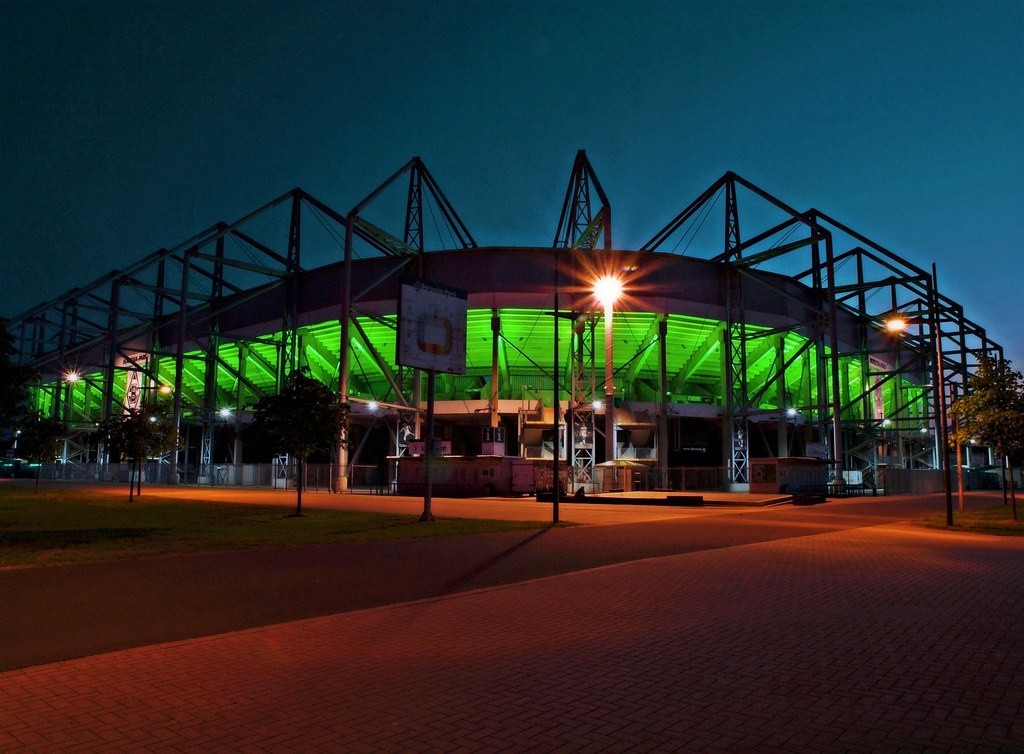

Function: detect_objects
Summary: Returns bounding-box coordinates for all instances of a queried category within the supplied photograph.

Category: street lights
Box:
[136,387,171,394]
[883,316,907,468]
[586,272,623,463]
[59,370,79,480]
